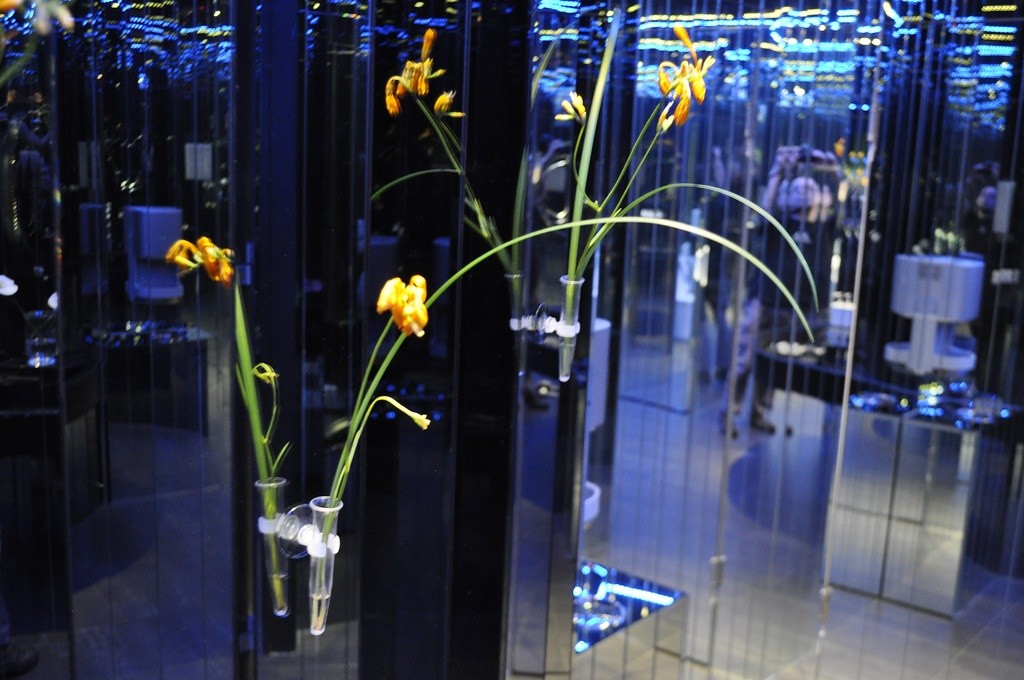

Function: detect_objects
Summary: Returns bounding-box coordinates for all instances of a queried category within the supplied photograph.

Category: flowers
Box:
[166,21,818,628]
